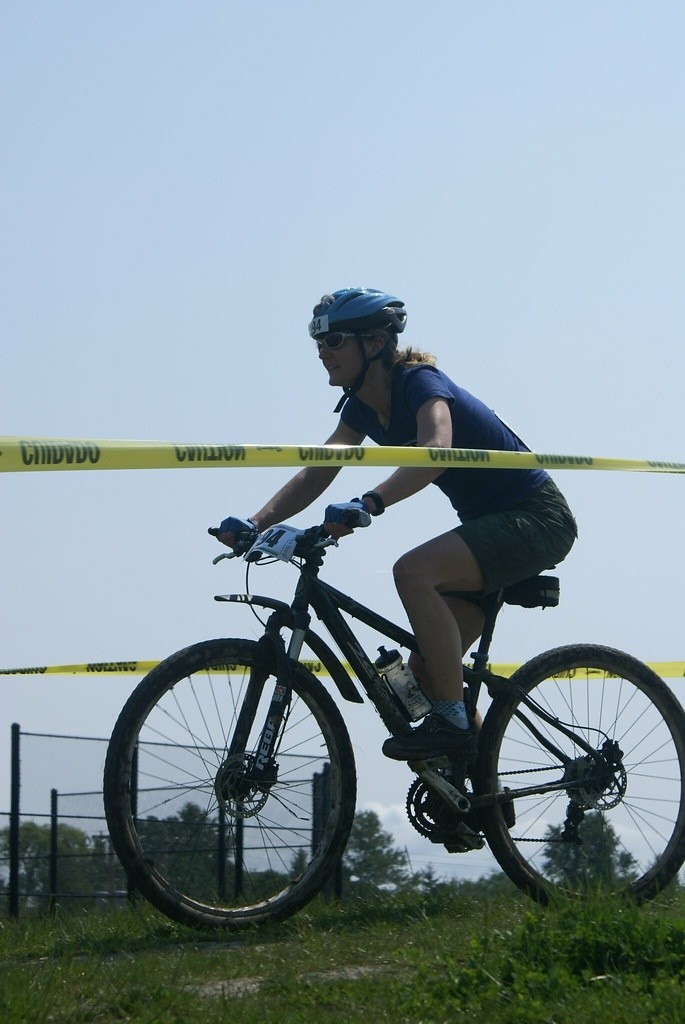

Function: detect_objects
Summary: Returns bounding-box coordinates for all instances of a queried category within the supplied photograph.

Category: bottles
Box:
[376,645,432,720]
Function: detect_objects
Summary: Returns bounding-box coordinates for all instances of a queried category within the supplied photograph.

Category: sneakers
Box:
[462,788,516,829]
[382,715,477,759]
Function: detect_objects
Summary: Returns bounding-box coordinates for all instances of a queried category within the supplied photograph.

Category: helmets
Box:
[310,289,406,359]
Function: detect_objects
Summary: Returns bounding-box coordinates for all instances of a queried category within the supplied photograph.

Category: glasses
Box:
[317,332,372,350]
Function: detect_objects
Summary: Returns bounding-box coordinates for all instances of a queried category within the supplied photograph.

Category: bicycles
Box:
[101,506,685,934]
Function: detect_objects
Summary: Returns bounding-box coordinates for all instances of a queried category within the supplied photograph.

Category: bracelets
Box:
[361,491,385,516]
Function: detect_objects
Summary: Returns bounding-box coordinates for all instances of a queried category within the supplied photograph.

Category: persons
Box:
[216,288,578,844]
